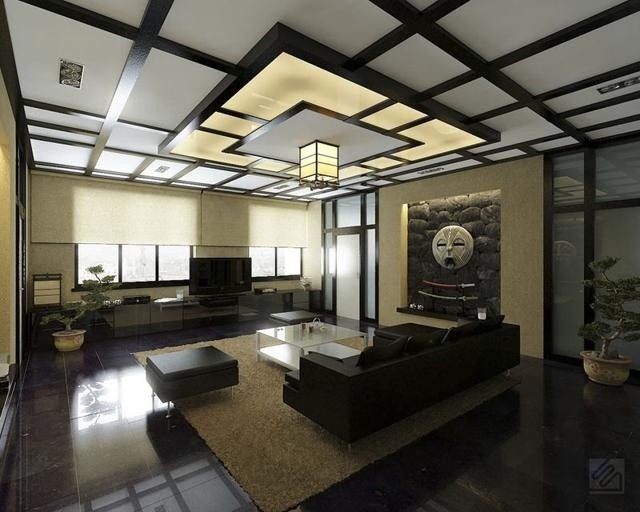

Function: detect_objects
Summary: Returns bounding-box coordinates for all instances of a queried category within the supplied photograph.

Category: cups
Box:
[302,323,314,333]
[176,289,183,301]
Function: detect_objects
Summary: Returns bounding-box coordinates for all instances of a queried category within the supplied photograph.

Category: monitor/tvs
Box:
[189,257,252,298]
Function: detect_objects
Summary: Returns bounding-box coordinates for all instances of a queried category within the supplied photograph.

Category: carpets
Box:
[131,329,521,512]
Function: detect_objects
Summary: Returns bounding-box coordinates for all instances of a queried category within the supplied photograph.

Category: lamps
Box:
[298,139,339,190]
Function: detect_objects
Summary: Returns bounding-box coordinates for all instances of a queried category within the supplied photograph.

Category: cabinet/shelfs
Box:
[99,283,323,340]
[29,306,116,349]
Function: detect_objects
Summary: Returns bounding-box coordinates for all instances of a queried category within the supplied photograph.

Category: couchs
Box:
[283,321,520,455]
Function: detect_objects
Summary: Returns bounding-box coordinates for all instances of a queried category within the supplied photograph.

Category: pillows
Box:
[355,314,505,369]
[309,351,344,363]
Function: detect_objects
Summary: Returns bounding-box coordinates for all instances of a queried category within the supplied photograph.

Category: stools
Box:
[268,309,323,325]
[146,345,239,431]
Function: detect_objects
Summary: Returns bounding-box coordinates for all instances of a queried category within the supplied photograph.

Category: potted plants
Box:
[38,264,123,352]
[576,257,639,388]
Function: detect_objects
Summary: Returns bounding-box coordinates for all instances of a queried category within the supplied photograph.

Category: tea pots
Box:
[311,317,322,332]
